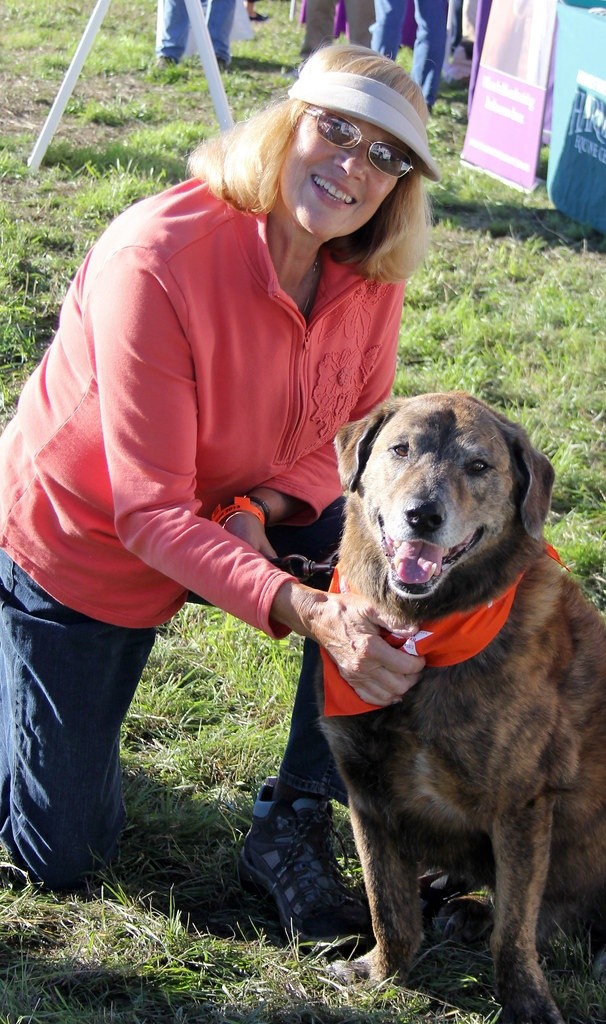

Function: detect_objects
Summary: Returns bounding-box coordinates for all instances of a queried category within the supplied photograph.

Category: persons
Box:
[150,0,481,115]
[0,40,445,948]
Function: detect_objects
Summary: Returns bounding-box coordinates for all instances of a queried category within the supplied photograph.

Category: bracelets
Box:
[210,496,265,528]
[225,494,271,527]
[220,511,251,532]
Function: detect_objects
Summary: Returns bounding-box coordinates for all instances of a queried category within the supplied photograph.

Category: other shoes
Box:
[249,13,270,22]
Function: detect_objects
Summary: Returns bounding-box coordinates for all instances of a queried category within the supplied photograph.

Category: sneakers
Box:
[237,777,371,948]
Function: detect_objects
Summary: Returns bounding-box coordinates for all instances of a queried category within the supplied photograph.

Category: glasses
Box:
[304,109,415,180]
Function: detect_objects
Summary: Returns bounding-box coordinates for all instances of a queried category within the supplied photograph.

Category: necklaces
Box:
[302,259,318,317]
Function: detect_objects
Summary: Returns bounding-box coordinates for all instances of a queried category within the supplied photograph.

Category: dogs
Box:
[315,390,605,1023]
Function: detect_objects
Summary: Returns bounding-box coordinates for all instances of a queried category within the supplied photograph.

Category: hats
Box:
[286,68,442,183]
